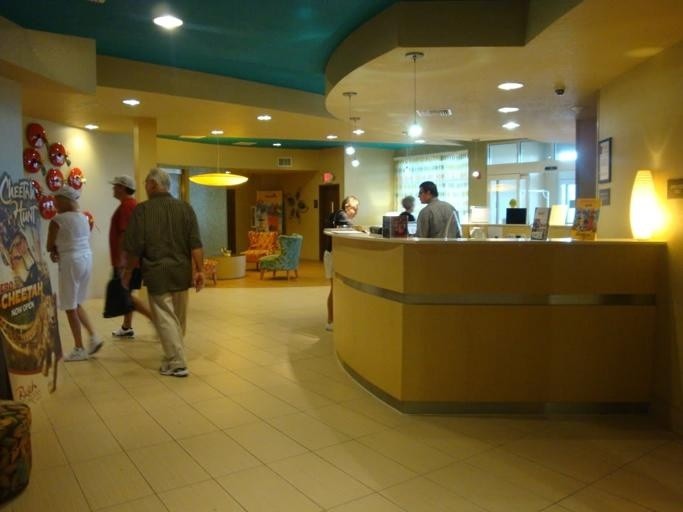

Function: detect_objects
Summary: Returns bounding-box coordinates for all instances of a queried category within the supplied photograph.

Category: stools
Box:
[0,399,32,502]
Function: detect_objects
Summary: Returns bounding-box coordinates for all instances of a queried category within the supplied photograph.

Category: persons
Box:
[399,195,416,222]
[109,176,152,337]
[409,181,463,238]
[47,184,104,362]
[121,167,205,377]
[322,195,370,333]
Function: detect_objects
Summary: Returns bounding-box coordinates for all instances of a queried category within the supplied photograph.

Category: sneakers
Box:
[63,336,102,361]
[160,366,188,376]
[112,326,133,336]
[326,323,333,331]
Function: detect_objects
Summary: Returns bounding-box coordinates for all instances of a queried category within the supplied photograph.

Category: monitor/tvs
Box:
[506,208,527,224]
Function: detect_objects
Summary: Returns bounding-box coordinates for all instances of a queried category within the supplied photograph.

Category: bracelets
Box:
[122,268,133,273]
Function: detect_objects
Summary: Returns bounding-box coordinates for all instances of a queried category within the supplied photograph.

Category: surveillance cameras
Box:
[552,84,566,96]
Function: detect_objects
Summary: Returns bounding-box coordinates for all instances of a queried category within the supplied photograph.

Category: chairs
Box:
[241,231,278,270]
[192,260,218,286]
[259,233,304,281]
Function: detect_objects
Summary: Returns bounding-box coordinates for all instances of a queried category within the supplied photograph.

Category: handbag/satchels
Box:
[103,279,135,317]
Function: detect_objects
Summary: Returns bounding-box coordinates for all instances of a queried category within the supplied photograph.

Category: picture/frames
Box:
[598,137,612,184]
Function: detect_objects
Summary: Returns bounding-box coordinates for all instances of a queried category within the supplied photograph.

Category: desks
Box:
[209,254,246,280]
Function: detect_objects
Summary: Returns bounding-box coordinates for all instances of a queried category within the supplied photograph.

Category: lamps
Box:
[188,135,248,186]
[404,52,424,138]
[342,92,356,155]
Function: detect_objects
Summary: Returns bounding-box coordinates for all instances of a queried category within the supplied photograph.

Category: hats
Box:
[111,176,135,191]
[50,187,80,200]
